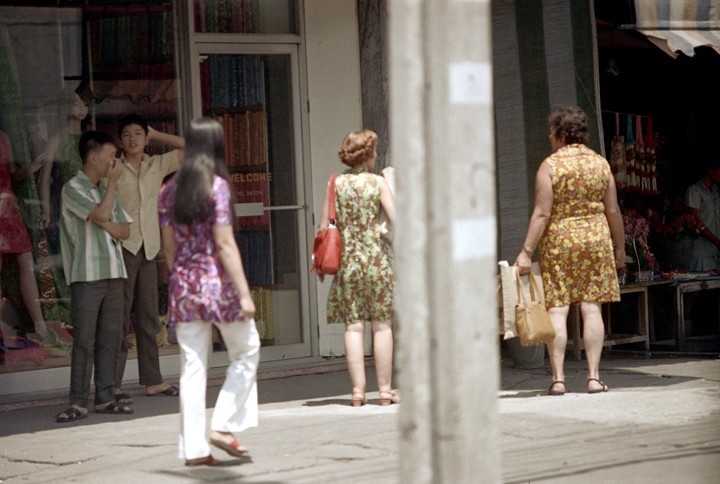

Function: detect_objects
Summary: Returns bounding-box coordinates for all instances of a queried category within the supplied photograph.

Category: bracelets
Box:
[522,244,535,255]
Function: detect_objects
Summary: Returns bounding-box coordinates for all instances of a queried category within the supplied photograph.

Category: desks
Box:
[650,280,720,353]
[567,287,650,361]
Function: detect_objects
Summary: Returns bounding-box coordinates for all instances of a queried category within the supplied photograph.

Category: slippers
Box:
[96,401,134,414]
[112,391,133,403]
[57,407,87,422]
[186,453,234,468]
[208,427,253,461]
[145,384,179,398]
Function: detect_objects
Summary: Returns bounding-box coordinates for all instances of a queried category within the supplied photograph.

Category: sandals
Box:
[352,392,368,406]
[548,381,567,396]
[378,389,400,405]
[587,378,610,393]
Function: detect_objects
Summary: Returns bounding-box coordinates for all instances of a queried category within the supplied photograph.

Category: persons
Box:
[510,106,629,396]
[157,114,263,465]
[310,129,400,410]
[55,129,133,426]
[100,117,189,406]
[682,154,720,276]
[0,81,92,352]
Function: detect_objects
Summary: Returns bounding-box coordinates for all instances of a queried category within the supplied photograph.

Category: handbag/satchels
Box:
[514,267,554,348]
[497,259,545,338]
[310,173,344,279]
